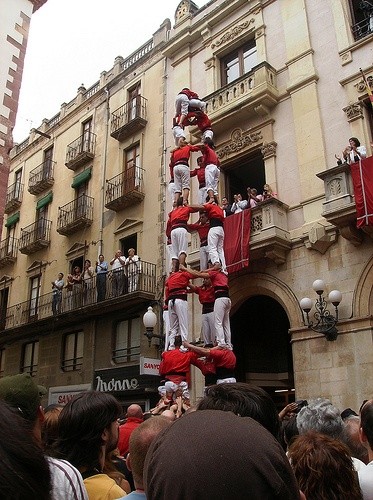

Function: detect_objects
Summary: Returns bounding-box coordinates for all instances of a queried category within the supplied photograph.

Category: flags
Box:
[359,67,373,108]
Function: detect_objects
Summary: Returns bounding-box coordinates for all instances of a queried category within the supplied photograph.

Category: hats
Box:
[144,411,300,500]
[1,373,48,410]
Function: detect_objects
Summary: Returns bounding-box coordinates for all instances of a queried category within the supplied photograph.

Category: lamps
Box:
[143,306,165,348]
[299,279,342,341]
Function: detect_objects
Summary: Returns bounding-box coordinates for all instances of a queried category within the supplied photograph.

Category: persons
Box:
[262,184,277,201]
[150,87,236,421]
[110,250,128,298]
[141,382,306,500]
[0,373,127,500]
[51,273,65,316]
[95,255,108,302]
[125,248,141,293]
[231,193,248,214]
[111,404,174,500]
[278,398,373,500]
[64,266,84,309]
[81,260,95,306]
[335,137,367,165]
[247,187,264,208]
[220,197,234,218]
